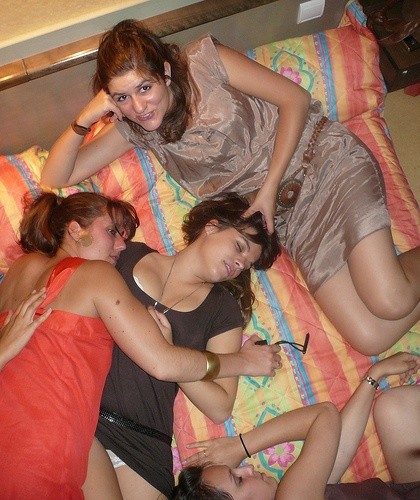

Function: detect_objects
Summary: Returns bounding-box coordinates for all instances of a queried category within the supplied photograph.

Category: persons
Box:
[40,18,420,355]
[0,188,282,500]
[80,193,284,500]
[0,287,52,371]
[169,352,420,500]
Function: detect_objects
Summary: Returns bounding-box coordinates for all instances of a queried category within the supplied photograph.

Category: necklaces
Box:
[153,254,205,314]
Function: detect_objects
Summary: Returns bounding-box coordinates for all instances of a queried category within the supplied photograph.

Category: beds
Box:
[0,0,420,484]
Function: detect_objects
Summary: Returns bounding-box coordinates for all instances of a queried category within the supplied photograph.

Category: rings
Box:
[203,449,207,458]
[277,362,281,369]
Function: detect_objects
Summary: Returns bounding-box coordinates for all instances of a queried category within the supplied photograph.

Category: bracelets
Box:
[71,120,92,135]
[239,433,252,458]
[201,351,220,382]
[362,375,381,390]
